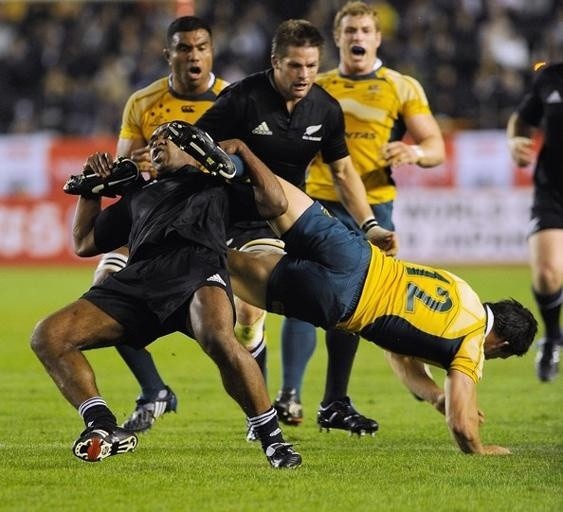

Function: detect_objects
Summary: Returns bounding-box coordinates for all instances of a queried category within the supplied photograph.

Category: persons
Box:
[93,16,271,441]
[505,61,562,383]
[63,120,538,455]
[192,18,399,441]
[271,0,447,438]
[31,121,303,470]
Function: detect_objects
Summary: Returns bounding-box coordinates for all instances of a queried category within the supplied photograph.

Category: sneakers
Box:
[537,340,560,381]
[167,120,236,180]
[63,159,139,193]
[72,387,177,462]
[266,390,378,469]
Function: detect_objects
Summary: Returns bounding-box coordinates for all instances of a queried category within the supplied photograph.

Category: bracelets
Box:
[414,144,425,163]
[359,216,379,235]
